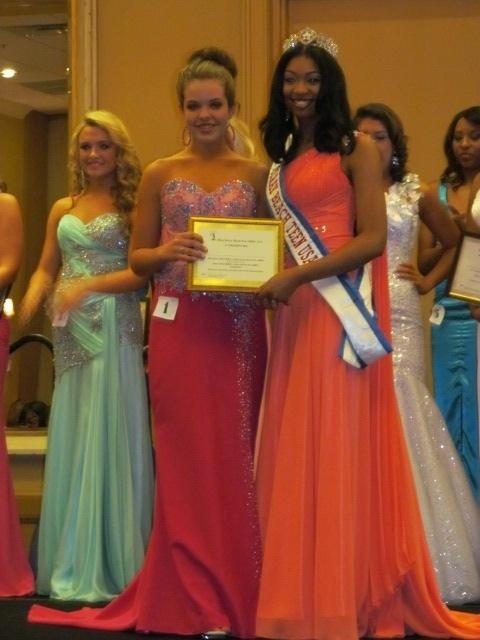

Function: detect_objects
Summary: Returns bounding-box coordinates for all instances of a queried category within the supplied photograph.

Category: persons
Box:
[355,102,480,605]
[28,48,269,640]
[0,111,155,600]
[250,30,480,639]
